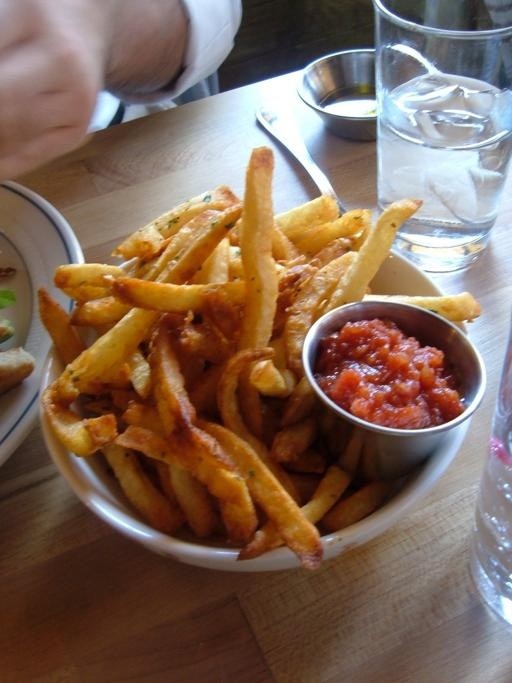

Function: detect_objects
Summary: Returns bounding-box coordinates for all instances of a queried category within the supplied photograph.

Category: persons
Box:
[0,0,243,180]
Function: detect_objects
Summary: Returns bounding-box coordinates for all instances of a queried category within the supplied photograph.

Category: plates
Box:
[1,177,88,468]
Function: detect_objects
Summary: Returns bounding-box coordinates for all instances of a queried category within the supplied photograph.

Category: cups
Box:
[300,300,491,481]
[370,0,512,274]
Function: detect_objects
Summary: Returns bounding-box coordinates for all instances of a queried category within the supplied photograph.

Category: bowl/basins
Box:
[34,218,483,577]
[296,49,434,141]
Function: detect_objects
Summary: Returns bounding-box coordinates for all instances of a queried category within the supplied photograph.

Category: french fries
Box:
[0,147,484,573]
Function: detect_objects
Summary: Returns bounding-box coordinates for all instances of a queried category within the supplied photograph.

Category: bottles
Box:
[475,283,509,635]
